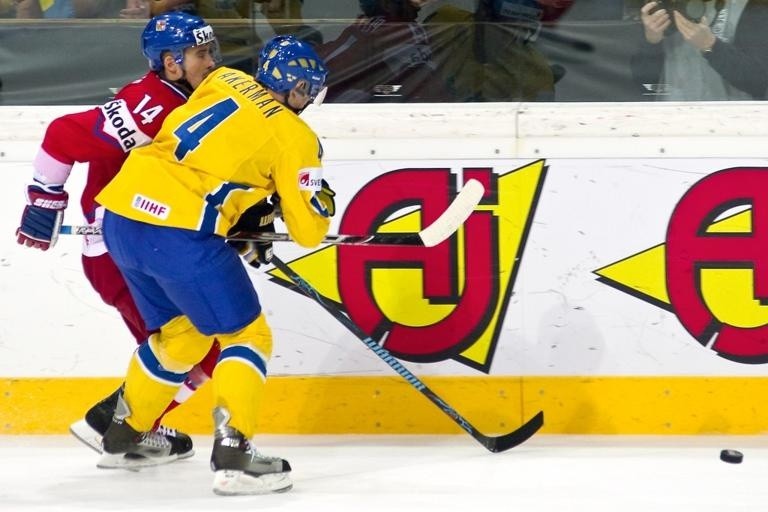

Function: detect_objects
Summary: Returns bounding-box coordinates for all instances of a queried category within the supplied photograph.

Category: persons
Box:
[93,33,330,477]
[1,0,767,101]
[16,11,274,455]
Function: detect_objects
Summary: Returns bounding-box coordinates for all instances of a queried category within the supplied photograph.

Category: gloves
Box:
[224,178,335,268]
[17,181,68,252]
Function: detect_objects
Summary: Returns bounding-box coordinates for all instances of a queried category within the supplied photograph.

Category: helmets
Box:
[141,10,223,73]
[254,34,328,103]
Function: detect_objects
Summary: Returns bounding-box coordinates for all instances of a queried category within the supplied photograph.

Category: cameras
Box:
[648,1,705,36]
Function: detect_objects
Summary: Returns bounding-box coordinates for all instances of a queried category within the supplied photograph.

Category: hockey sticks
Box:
[59,178,486,249]
[272,254,543,452]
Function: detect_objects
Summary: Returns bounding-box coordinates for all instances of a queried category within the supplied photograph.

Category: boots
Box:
[83,382,193,455]
[210,405,292,476]
